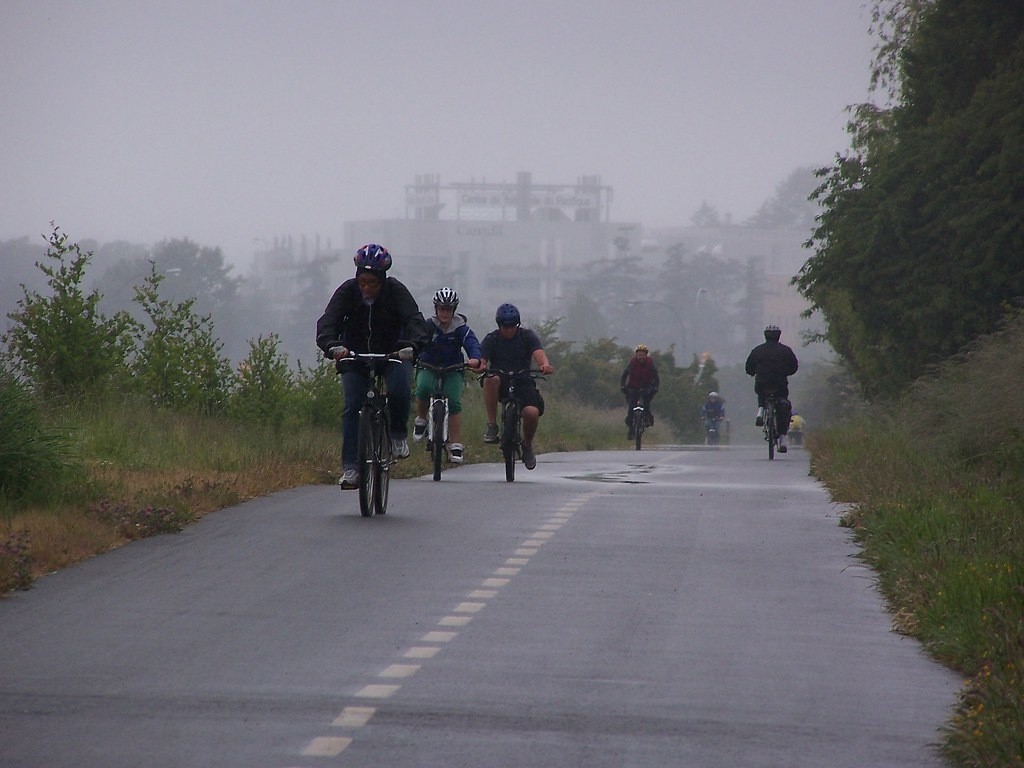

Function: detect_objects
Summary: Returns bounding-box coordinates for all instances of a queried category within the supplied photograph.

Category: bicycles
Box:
[413,357,475,481]
[702,415,722,446]
[790,430,801,445]
[754,372,785,460]
[326,347,418,518]
[481,367,545,482]
[622,385,658,451]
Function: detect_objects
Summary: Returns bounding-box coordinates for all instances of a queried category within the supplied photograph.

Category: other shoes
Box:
[780,445,787,452]
[627,428,635,440]
[521,440,536,470]
[755,416,763,426]
[483,422,499,442]
[450,442,465,463]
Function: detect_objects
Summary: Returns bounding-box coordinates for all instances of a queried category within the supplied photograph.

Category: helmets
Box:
[432,287,459,308]
[708,392,719,399]
[353,243,392,273]
[791,409,798,414]
[635,345,648,353]
[496,303,520,327]
[764,324,780,332]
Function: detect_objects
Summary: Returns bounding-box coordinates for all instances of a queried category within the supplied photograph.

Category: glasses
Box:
[439,307,453,313]
[357,274,378,288]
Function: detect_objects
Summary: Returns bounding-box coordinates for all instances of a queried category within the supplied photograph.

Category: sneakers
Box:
[413,416,430,442]
[392,437,410,459]
[338,468,361,487]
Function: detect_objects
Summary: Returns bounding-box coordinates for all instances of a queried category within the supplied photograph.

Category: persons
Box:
[745,325,798,452]
[473,304,554,470]
[413,287,480,463]
[787,408,807,449]
[316,244,426,487]
[701,392,725,445]
[620,345,660,439]
